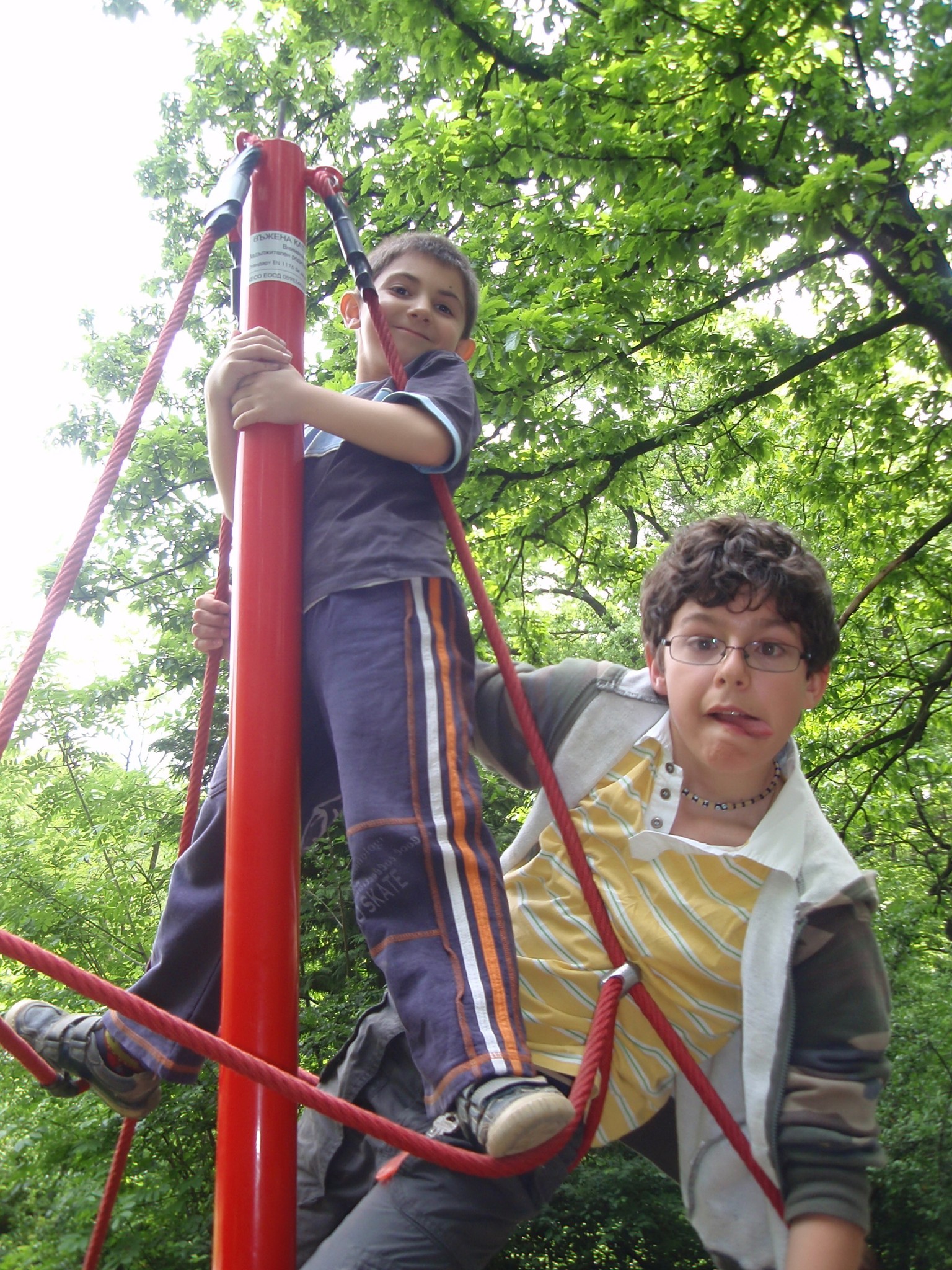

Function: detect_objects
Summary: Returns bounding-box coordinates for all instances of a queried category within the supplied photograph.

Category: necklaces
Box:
[682,758,783,812]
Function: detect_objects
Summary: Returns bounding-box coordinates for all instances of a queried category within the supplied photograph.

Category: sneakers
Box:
[454,1074,575,1156]
[4,996,162,1119]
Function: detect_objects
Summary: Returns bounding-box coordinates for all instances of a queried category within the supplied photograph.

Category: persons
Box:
[3,233,575,1157]
[193,521,888,1270]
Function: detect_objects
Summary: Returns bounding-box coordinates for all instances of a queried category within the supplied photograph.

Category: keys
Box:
[424,1110,462,1138]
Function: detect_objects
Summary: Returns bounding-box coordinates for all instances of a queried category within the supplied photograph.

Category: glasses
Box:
[656,633,812,674]
